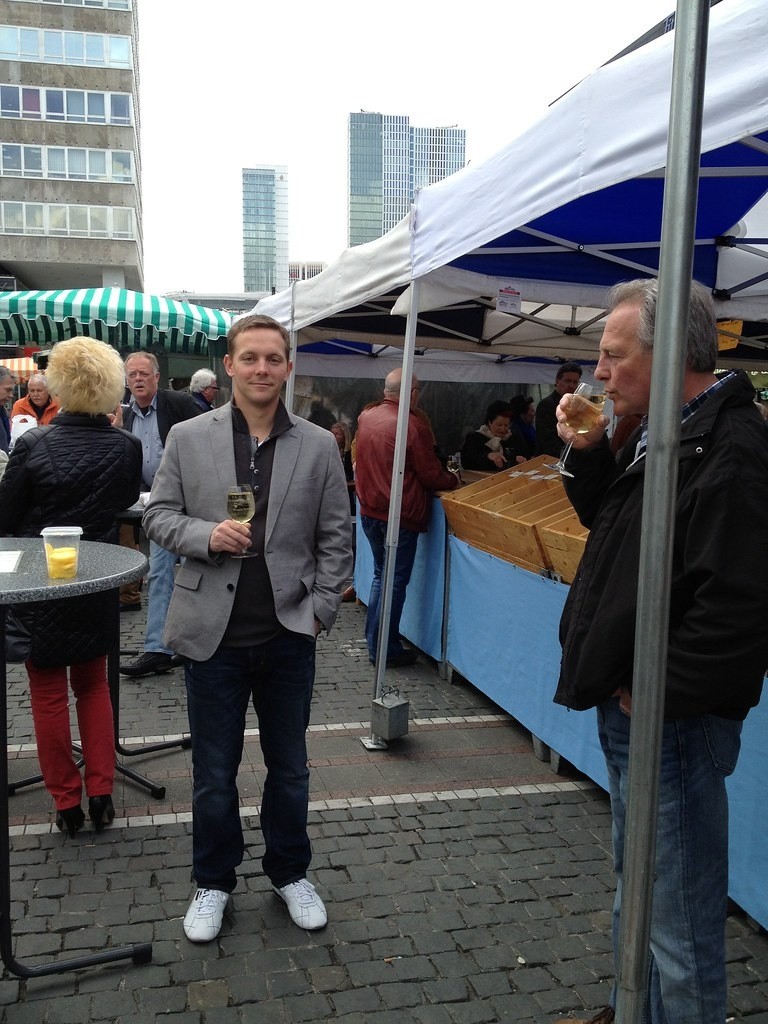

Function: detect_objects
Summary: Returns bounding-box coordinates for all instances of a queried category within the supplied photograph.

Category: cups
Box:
[447,461,460,473]
[39,526,83,578]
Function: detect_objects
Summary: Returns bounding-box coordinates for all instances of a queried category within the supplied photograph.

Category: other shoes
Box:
[370,648,420,669]
[120,603,143,613]
[555,1006,615,1024]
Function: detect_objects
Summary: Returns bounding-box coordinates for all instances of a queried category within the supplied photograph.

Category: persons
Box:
[534,362,583,459]
[509,394,535,461]
[0,336,143,840]
[519,276,768,1023]
[9,373,65,432]
[141,313,354,943]
[119,351,205,677]
[460,399,527,471]
[355,367,461,669]
[0,366,17,482]
[119,517,143,612]
[190,368,220,412]
[330,421,354,482]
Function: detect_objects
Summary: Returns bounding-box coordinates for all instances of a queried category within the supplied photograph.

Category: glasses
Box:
[210,386,220,392]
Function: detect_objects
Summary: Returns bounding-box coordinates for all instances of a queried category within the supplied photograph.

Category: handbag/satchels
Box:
[9,414,37,448]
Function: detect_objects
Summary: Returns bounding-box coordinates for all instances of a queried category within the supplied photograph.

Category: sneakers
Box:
[272,879,329,931]
[183,887,232,944]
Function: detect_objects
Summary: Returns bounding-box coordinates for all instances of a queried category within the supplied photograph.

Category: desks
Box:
[446,535,768,933]
[6,491,191,805]
[0,536,155,978]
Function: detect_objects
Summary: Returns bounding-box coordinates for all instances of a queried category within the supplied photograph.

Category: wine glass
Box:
[225,484,258,558]
[542,381,607,477]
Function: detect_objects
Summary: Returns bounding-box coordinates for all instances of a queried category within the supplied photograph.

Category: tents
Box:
[0,0,768,1024]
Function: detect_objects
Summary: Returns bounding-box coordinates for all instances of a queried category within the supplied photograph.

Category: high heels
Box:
[56,805,86,838]
[89,795,115,832]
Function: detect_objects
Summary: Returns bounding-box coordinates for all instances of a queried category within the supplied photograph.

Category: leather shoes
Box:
[120,653,184,677]
